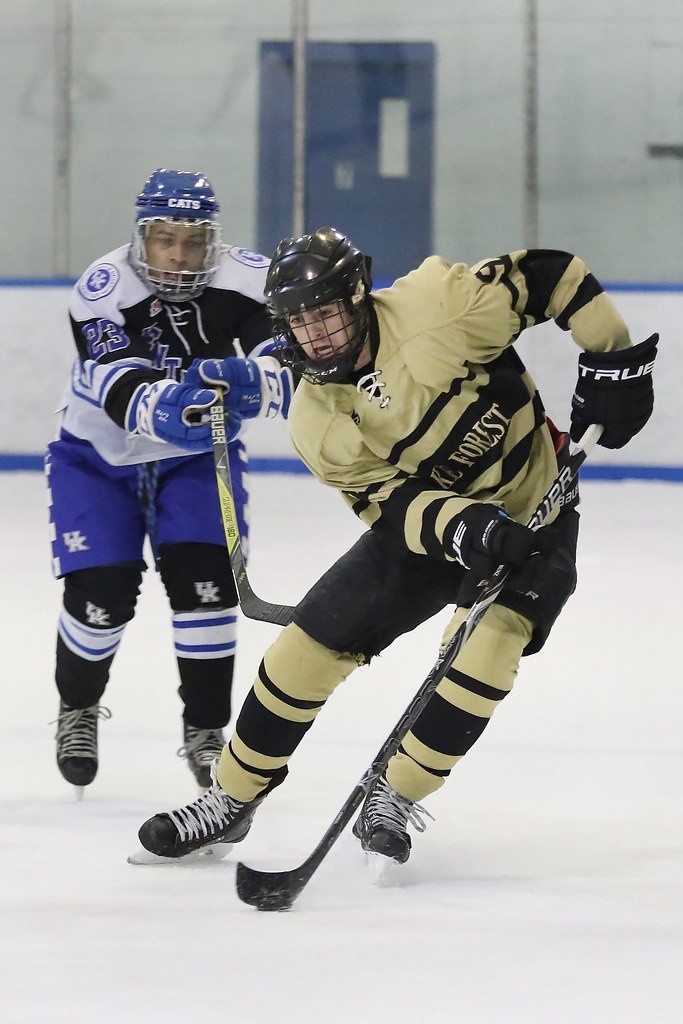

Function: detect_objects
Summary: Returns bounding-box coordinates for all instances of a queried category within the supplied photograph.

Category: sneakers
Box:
[352,762,435,880]
[127,757,287,865]
[47,698,112,801]
[176,720,227,798]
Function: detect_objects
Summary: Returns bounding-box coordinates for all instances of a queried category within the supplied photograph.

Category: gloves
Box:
[180,354,294,421]
[569,333,661,450]
[443,502,552,584]
[123,378,246,454]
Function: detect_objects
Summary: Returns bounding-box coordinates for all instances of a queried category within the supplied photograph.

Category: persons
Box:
[127,226,659,887]
[44,168,300,801]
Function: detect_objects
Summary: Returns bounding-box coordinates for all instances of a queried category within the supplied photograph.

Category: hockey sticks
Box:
[211,385,295,627]
[234,423,601,915]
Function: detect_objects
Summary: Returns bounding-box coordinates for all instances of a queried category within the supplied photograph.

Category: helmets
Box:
[262,225,374,386]
[127,167,225,303]
